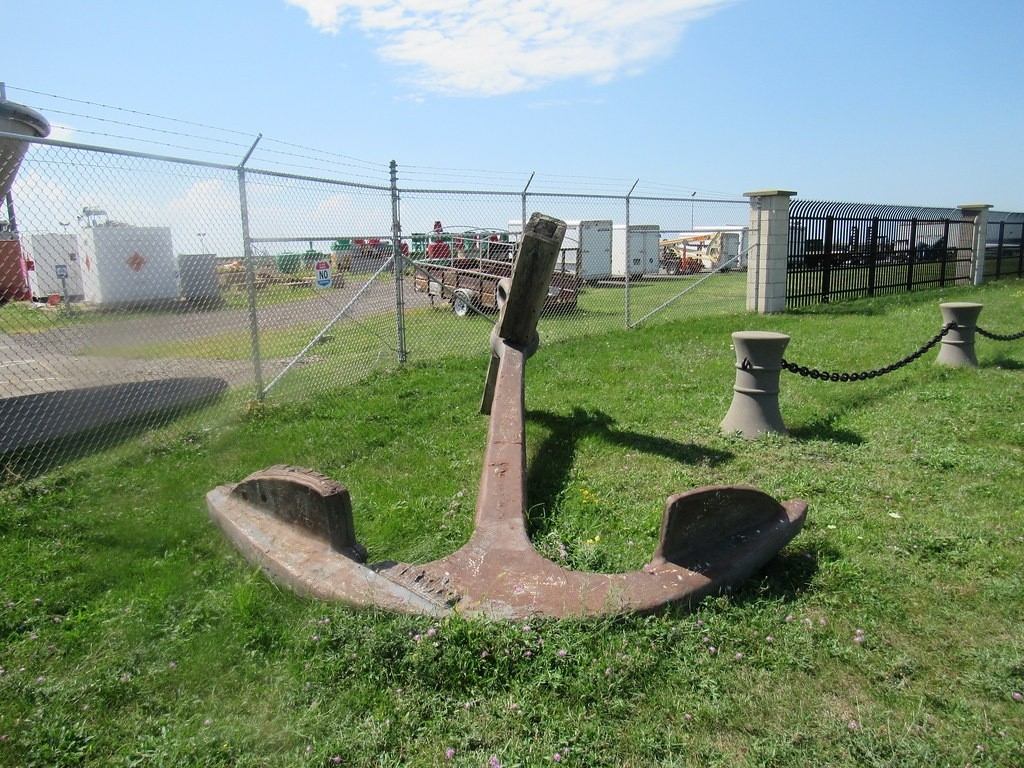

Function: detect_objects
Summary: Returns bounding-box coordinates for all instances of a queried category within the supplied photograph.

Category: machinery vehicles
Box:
[657,234,711,276]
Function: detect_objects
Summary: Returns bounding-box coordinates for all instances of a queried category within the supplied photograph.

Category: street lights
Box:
[691,191,696,230]
